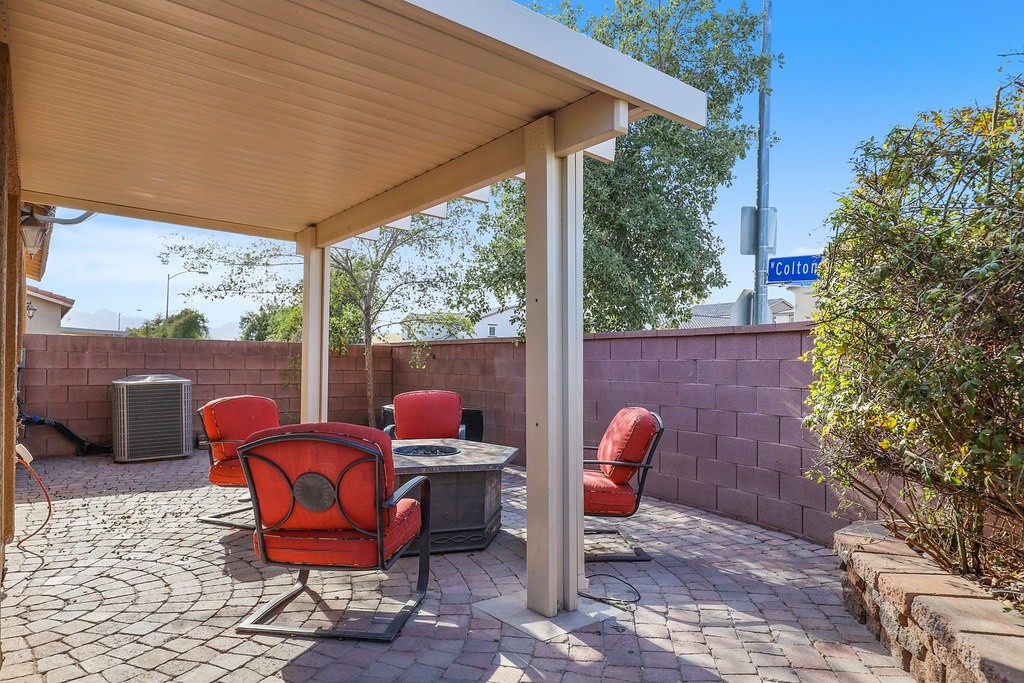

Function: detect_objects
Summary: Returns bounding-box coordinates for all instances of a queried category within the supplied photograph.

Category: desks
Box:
[391,438,519,555]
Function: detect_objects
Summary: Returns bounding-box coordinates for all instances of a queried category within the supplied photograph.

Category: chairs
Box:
[235,421,432,641]
[584,407,665,562]
[196,395,279,529]
[383,389,465,440]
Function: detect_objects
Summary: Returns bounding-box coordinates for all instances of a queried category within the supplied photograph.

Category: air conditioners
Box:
[112,374,195,460]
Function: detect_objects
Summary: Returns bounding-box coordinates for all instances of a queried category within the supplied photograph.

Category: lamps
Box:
[26,299,37,320]
[19,205,46,260]
